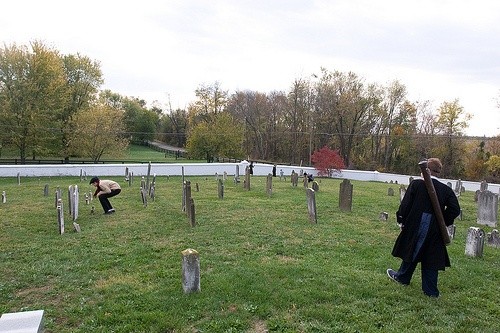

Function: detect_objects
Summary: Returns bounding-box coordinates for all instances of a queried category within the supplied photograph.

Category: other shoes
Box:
[387,269,404,286]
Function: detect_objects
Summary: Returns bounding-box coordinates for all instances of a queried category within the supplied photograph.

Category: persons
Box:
[89,176,122,214]
[249,161,255,174]
[386,157,460,299]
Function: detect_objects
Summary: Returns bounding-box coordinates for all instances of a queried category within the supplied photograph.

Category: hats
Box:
[89,177,98,184]
